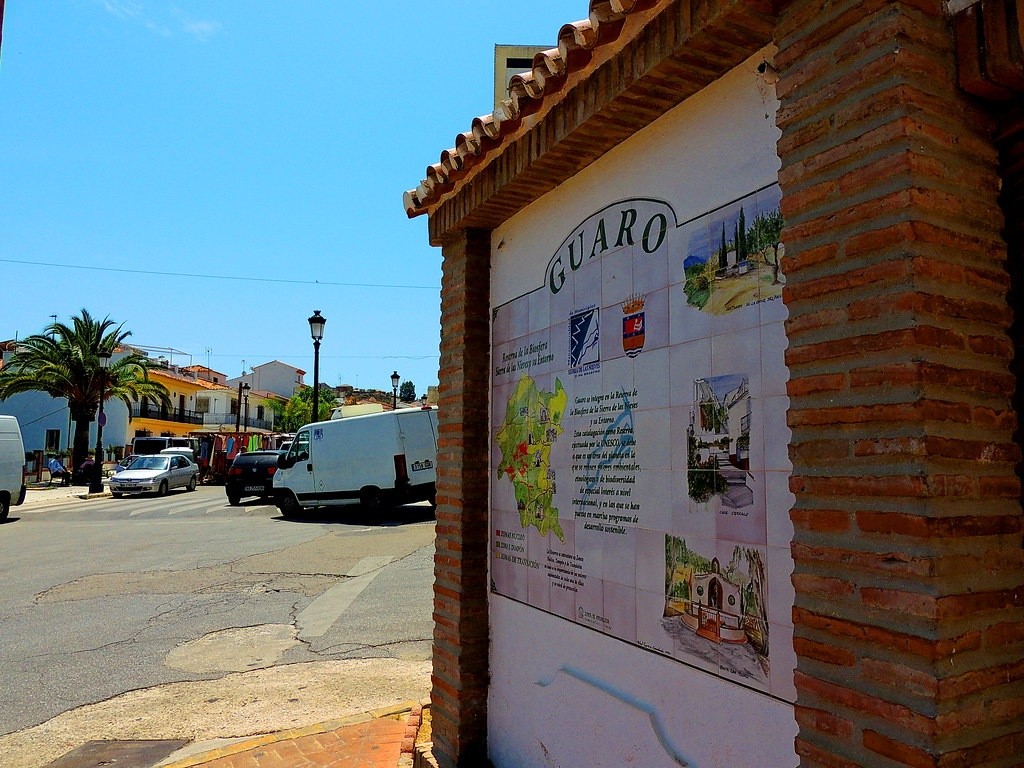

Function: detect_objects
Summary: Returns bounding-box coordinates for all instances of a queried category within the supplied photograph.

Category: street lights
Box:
[89,346,113,493]
[306,308,328,423]
[49,314,58,325]
[389,369,401,411]
[242,382,251,433]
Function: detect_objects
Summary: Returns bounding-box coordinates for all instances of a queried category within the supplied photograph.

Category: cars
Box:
[115,454,153,473]
[108,455,201,499]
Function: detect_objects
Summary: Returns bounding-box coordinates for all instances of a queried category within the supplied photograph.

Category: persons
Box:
[78,456,95,472]
[49,455,71,487]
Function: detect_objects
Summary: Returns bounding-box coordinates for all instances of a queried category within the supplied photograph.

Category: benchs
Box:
[46,466,71,486]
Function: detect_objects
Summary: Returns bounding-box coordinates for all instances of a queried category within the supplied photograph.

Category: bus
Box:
[131,435,201,459]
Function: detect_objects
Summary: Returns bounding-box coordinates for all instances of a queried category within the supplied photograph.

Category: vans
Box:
[0,414,27,522]
[272,405,439,523]
[278,440,310,458]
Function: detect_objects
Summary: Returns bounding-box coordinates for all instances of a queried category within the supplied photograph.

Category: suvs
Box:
[223,448,295,506]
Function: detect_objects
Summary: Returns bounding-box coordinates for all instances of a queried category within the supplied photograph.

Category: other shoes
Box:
[66,484,72,487]
